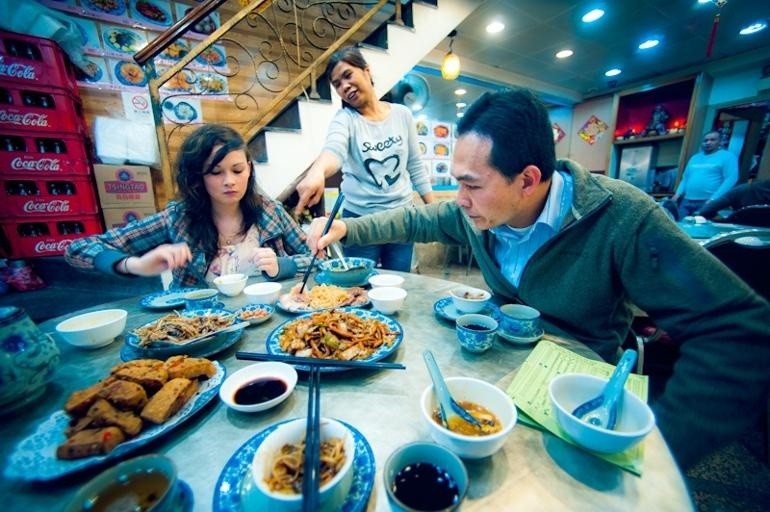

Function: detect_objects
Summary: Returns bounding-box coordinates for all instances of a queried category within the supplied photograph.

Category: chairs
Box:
[726,205,770,228]
[672,232,769,512]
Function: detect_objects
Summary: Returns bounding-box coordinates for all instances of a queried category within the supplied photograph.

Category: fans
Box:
[387,72,431,115]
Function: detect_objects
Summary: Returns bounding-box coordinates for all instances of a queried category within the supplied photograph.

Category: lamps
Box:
[439,36,460,81]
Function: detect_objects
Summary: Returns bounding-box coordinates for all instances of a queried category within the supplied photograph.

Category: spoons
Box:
[145,321,250,348]
[422,349,480,434]
[572,348,638,432]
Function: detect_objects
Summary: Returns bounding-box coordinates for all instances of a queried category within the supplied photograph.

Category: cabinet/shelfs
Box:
[605,72,711,198]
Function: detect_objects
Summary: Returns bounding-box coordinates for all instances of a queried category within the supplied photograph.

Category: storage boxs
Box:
[0,174,97,216]
[101,206,158,229]
[0,82,85,133]
[1,30,83,97]
[1,134,90,175]
[1,216,103,257]
[93,161,156,208]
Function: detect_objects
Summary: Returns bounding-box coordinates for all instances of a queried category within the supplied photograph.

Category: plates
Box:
[211,415,375,512]
[126,309,234,352]
[131,2,173,26]
[497,325,545,346]
[196,46,225,66]
[185,7,216,35]
[195,73,227,95]
[433,296,502,323]
[313,270,380,289]
[276,290,372,313]
[103,27,145,52]
[2,357,225,484]
[264,308,404,373]
[234,303,276,325]
[109,59,148,88]
[176,478,195,512]
[120,308,244,364]
[83,56,105,84]
[83,0,126,16]
[158,68,192,93]
[139,288,199,309]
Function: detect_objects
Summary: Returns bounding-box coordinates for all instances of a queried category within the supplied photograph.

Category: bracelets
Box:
[125,257,129,273]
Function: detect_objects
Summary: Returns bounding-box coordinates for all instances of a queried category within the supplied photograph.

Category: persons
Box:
[65,123,328,290]
[306,89,770,470]
[295,46,434,273]
[671,131,739,221]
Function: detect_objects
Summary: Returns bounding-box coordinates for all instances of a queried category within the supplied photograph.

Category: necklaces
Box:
[218,225,245,245]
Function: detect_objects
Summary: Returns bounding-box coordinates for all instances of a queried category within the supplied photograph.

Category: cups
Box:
[183,289,218,311]
[499,304,541,336]
[455,313,498,356]
[383,440,468,511]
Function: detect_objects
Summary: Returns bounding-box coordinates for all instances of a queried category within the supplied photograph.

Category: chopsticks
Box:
[234,350,406,370]
[185,260,209,289]
[301,363,321,511]
[299,192,345,295]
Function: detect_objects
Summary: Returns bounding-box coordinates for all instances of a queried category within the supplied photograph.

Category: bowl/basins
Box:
[448,287,492,313]
[421,376,518,460]
[213,273,248,297]
[218,361,298,414]
[244,281,282,305]
[55,308,128,351]
[368,274,405,289]
[547,373,657,454]
[59,454,179,512]
[367,287,407,315]
[250,416,355,501]
[316,256,376,283]
